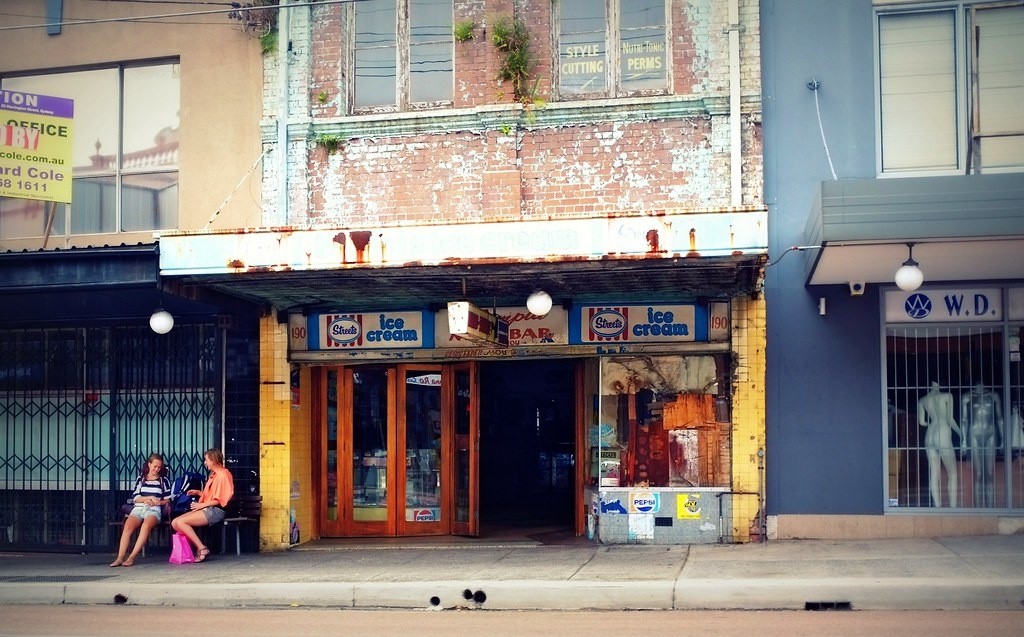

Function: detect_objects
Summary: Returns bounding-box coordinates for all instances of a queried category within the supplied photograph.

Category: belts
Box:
[217,505,227,511]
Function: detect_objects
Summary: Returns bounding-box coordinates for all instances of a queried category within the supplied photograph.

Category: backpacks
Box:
[171,472,206,512]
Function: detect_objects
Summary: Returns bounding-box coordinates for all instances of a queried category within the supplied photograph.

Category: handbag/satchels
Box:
[168,532,195,563]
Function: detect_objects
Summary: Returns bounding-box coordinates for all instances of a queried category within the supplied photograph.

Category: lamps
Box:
[895,242,923,291]
[526,288,552,315]
[150,296,174,334]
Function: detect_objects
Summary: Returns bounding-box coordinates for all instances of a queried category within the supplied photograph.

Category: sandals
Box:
[123,556,136,566]
[110,559,123,566]
[194,546,211,562]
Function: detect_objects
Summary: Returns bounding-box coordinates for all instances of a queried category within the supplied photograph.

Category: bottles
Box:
[191,498,197,512]
[290,506,296,523]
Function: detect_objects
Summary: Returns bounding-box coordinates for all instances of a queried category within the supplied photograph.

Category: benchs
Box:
[109,495,263,557]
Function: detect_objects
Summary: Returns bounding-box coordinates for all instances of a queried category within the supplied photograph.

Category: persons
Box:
[109,453,172,568]
[170,448,234,563]
[918,374,1004,508]
